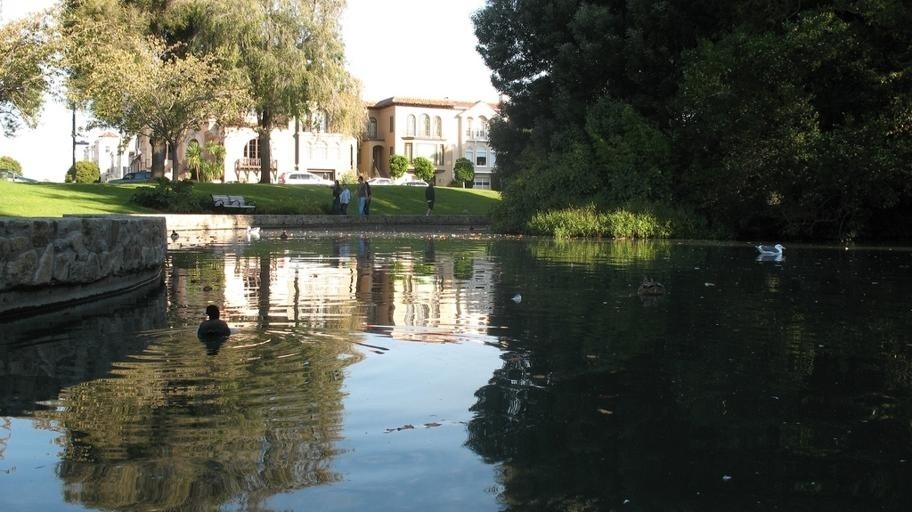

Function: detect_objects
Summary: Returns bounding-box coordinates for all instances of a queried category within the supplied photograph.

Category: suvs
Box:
[108,170,169,184]
[278,171,335,185]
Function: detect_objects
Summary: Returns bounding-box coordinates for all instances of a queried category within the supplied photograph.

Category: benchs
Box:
[210,193,257,215]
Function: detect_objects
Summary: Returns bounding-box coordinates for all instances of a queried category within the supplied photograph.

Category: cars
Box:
[400,181,429,186]
[356,177,394,186]
[1,169,39,183]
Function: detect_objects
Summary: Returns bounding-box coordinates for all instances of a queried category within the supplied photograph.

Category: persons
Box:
[339,184,350,214]
[358,176,368,216]
[425,182,434,216]
[425,240,436,263]
[332,181,341,211]
[197,305,230,338]
[332,237,369,268]
[363,182,372,215]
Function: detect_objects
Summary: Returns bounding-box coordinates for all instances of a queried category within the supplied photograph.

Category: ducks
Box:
[171,229,179,239]
[198,303,231,345]
[247,225,261,232]
[637,273,667,298]
[754,242,787,255]
[280,229,288,238]
